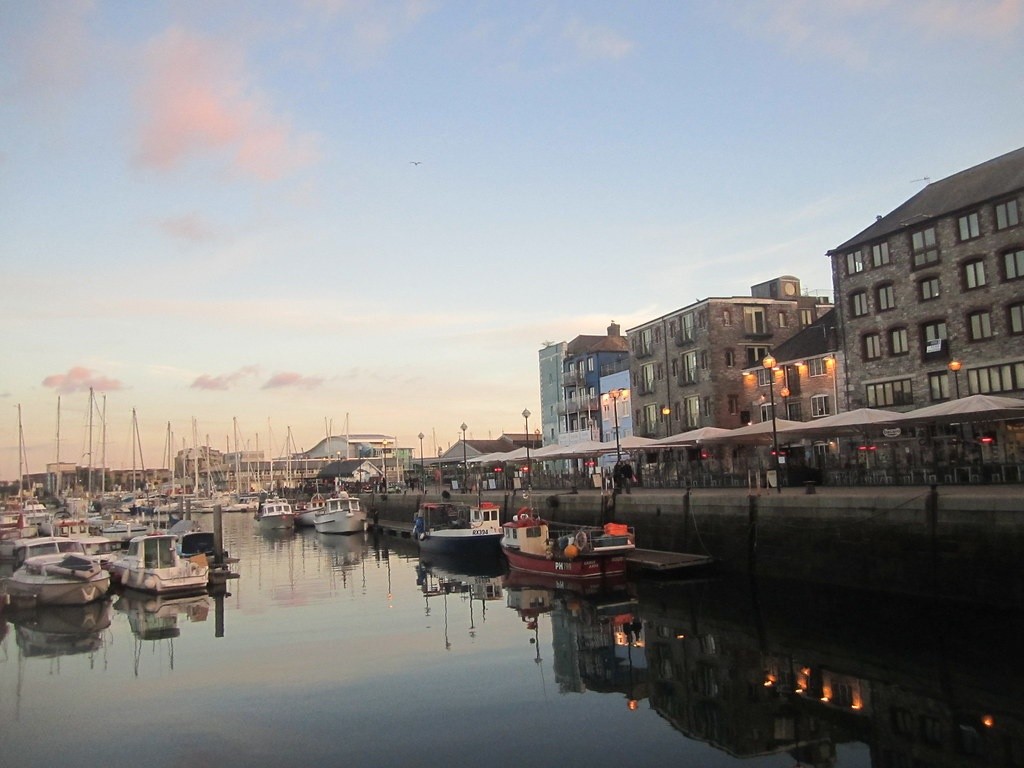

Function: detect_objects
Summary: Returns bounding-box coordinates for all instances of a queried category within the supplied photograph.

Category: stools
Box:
[734,480,741,486]
[992,473,1000,484]
[713,480,721,487]
[929,474,936,484]
[902,474,910,484]
[944,474,953,484]
[693,481,700,488]
[886,475,894,484]
[972,473,982,484]
[680,481,686,488]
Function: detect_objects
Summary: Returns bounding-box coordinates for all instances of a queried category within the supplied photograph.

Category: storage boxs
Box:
[58,555,92,571]
[604,523,627,535]
[190,553,208,567]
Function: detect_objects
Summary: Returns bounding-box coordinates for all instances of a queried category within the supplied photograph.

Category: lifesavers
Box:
[573,531,588,550]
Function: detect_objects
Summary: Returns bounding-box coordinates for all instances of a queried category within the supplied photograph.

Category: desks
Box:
[1001,463,1024,482]
[874,469,896,484]
[911,466,934,484]
[724,474,740,487]
[684,475,699,488]
[702,472,722,487]
[954,465,979,483]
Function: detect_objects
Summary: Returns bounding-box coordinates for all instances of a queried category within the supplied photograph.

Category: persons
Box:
[614,459,632,494]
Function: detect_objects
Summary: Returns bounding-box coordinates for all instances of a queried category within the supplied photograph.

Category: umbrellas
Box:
[459,426,732,477]
[872,395,1024,443]
[776,408,904,469]
[696,417,805,449]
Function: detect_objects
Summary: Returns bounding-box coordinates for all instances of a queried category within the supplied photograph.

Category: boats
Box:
[500,563,638,665]
[412,546,503,647]
[0,387,373,608]
[410,502,513,552]
[10,599,115,662]
[497,502,638,579]
[111,589,213,676]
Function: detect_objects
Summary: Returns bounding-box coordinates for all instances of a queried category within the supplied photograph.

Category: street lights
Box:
[382,439,388,495]
[418,433,424,493]
[460,422,467,493]
[588,418,594,442]
[658,406,671,437]
[608,389,621,462]
[522,409,532,489]
[762,351,784,492]
[337,451,341,488]
[949,361,961,400]
